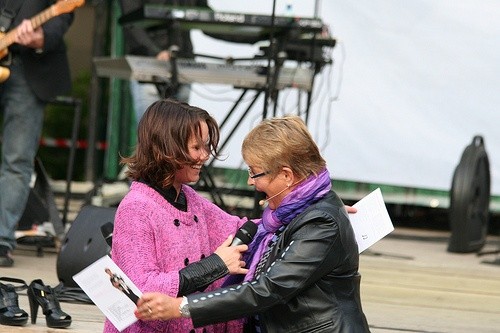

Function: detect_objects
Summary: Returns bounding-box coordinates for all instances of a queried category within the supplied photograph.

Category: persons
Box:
[119,0,213,103]
[134,115,371,333]
[0,0,76,267]
[104,99,357,333]
[104,268,139,307]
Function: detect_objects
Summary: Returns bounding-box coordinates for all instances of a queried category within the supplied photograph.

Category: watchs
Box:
[179,296,192,319]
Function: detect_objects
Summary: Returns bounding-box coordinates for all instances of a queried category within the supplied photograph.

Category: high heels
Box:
[0,276,29,326]
[27,279,71,328]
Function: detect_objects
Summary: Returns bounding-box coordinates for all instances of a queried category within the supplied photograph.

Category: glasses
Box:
[248,166,289,179]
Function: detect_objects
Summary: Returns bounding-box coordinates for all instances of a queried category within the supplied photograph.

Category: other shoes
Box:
[0,245,12,267]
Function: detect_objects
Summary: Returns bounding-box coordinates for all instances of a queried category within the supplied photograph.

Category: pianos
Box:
[111,1,326,34]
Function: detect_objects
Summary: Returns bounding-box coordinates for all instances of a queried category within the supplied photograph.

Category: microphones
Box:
[100,222,114,247]
[259,187,288,205]
[230,220,258,246]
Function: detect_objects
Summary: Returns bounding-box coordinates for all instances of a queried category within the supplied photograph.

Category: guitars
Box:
[0,1,88,84]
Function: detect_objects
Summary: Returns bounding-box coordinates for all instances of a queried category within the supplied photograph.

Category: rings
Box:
[147,303,151,308]
[148,310,152,316]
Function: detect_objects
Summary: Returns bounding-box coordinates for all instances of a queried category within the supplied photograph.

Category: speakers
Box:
[56,204,117,282]
